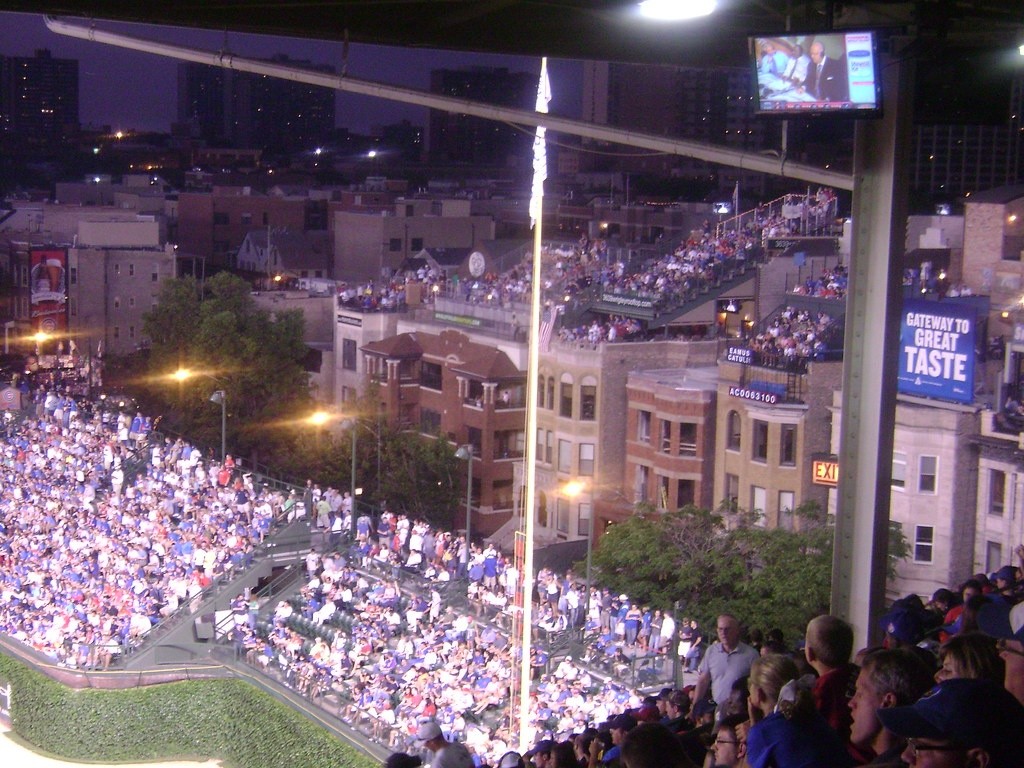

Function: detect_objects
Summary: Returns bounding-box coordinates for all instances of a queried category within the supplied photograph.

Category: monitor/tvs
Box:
[747,27,883,120]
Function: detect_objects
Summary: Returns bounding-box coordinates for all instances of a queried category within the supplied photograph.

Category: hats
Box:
[875,678,1024,761]
[529,740,558,756]
[939,605,977,635]
[630,704,660,722]
[641,696,657,704]
[692,700,716,725]
[764,628,784,642]
[412,723,442,748]
[660,689,691,708]
[993,566,1017,580]
[645,688,673,700]
[497,751,522,768]
[601,714,637,732]
[977,600,1024,641]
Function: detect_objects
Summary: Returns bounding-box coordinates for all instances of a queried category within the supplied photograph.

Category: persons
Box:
[602,186,838,309]
[793,258,849,301]
[558,312,749,349]
[749,305,835,375]
[461,233,607,307]
[994,374,1024,434]
[756,42,844,101]
[902,257,971,297]
[1,354,295,673]
[230,479,700,768]
[339,266,450,312]
[619,546,1024,768]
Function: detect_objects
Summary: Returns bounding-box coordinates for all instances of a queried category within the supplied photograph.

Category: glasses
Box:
[902,737,946,761]
[714,739,741,751]
[996,638,1024,657]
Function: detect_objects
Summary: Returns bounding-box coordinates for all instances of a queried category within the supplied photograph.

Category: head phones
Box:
[815,41,825,57]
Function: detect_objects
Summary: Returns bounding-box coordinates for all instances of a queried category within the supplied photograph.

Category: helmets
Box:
[879,607,925,643]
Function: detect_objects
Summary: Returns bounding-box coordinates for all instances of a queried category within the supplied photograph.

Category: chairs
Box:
[251,584,364,654]
